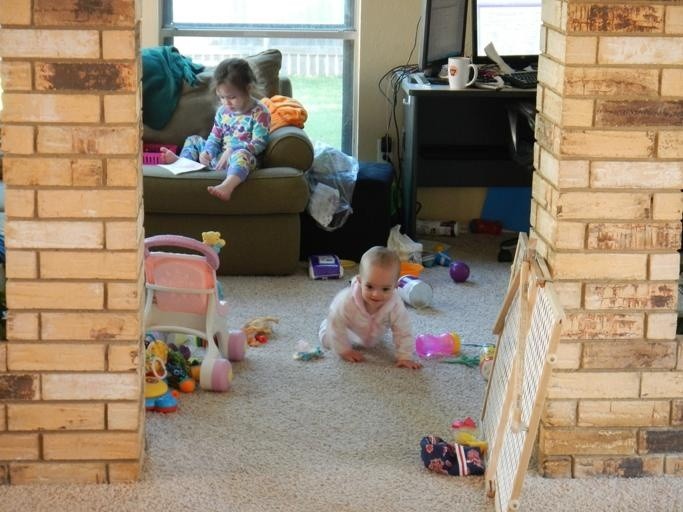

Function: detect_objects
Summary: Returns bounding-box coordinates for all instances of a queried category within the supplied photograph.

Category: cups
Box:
[396,275,434,309]
[447,56,479,90]
[478,342,497,380]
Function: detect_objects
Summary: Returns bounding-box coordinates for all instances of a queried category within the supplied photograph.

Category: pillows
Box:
[142,49,281,144]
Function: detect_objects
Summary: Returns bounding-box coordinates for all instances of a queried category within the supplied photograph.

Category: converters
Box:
[382,137,391,152]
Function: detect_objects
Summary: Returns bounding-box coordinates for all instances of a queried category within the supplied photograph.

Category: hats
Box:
[420,436,484,477]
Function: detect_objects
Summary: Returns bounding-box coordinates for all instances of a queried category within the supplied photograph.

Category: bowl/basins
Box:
[400,262,424,279]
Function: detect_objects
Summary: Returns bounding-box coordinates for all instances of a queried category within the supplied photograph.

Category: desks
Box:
[396,68,537,240]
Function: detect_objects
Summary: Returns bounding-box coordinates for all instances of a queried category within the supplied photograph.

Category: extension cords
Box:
[377,139,392,163]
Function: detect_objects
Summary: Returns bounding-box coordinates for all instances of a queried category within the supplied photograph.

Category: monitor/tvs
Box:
[471,0,542,71]
[418,0,468,85]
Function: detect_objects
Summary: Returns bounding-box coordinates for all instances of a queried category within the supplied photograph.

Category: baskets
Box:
[143,144,177,165]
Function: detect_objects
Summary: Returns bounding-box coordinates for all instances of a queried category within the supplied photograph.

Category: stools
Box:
[313,161,394,262]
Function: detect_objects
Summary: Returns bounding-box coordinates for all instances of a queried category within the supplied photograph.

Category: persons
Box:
[315,245,427,370]
[158,57,271,200]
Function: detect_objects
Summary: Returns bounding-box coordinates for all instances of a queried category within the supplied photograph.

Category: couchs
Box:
[142,49,313,277]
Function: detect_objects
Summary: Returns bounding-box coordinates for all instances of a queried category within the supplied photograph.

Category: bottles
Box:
[415,332,461,360]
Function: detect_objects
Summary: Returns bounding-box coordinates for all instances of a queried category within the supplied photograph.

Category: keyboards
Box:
[477,72,537,84]
